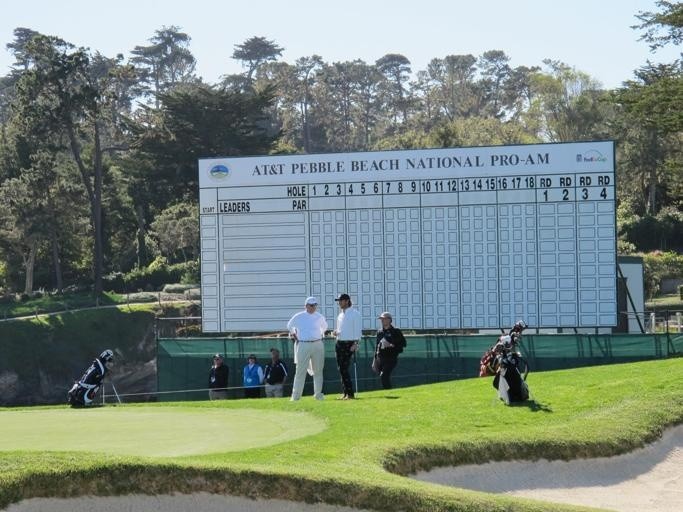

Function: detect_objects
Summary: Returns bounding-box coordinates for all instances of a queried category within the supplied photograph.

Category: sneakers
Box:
[336,393,355,400]
[315,392,325,400]
[290,393,299,401]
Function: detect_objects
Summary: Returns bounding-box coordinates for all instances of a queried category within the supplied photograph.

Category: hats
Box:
[305,297,319,306]
[271,348,279,352]
[335,293,349,300]
[213,354,223,357]
[377,312,392,319]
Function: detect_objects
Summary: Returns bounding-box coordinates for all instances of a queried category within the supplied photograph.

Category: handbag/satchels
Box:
[497,353,529,404]
[371,344,381,375]
[67,357,108,406]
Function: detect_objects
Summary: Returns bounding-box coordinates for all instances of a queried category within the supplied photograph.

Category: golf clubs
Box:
[99,350,121,404]
[355,340,358,399]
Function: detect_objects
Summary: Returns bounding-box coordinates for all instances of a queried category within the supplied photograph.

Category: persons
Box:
[208,354,229,400]
[264,348,289,399]
[285,295,328,402]
[330,293,364,400]
[374,311,407,390]
[242,354,264,399]
